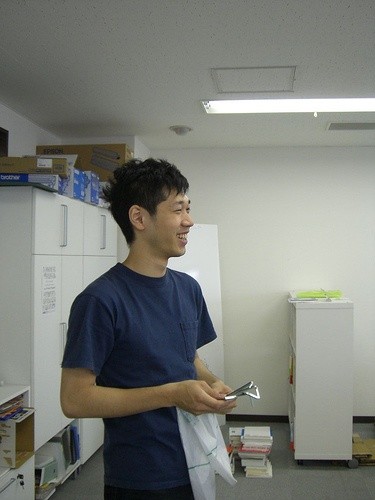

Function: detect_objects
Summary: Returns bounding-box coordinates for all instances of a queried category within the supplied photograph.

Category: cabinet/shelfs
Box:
[0,185,141,500]
[288,299,354,466]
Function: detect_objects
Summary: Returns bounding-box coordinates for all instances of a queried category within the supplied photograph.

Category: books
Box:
[351,432,375,464]
[215,426,273,479]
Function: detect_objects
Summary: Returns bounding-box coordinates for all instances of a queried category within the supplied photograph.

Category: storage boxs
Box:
[0,143,131,208]
[35,455,57,487]
[0,408,34,469]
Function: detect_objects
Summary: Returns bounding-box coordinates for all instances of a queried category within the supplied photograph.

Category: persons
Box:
[59,157,238,500]
